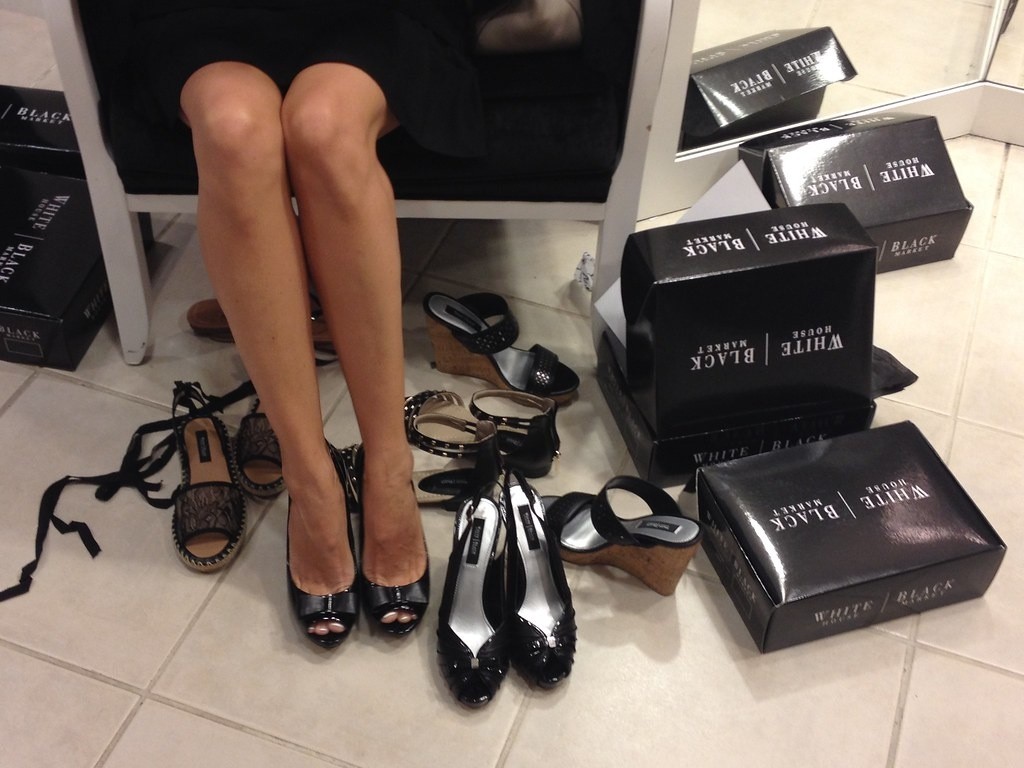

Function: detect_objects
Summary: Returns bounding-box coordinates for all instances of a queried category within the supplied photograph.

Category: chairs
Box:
[45,0,673,366]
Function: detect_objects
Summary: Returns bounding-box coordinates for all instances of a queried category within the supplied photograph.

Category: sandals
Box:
[407,386,561,478]
[234,395,287,496]
[336,414,499,511]
[167,379,247,570]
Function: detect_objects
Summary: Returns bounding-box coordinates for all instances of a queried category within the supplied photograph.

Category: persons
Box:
[128,0,580,649]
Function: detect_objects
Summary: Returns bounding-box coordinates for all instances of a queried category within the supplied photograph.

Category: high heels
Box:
[424,292,579,405]
[352,438,430,635]
[499,467,577,685]
[437,480,508,707]
[540,474,704,596]
[286,437,361,648]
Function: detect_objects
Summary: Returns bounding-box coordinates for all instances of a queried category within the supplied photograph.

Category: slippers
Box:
[187,290,335,344]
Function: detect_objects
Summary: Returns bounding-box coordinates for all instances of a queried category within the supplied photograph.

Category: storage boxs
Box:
[677,26,859,153]
[0,85,154,372]
[592,112,1008,656]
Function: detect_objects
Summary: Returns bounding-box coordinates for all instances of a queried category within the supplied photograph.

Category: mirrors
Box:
[669,0,1024,166]
